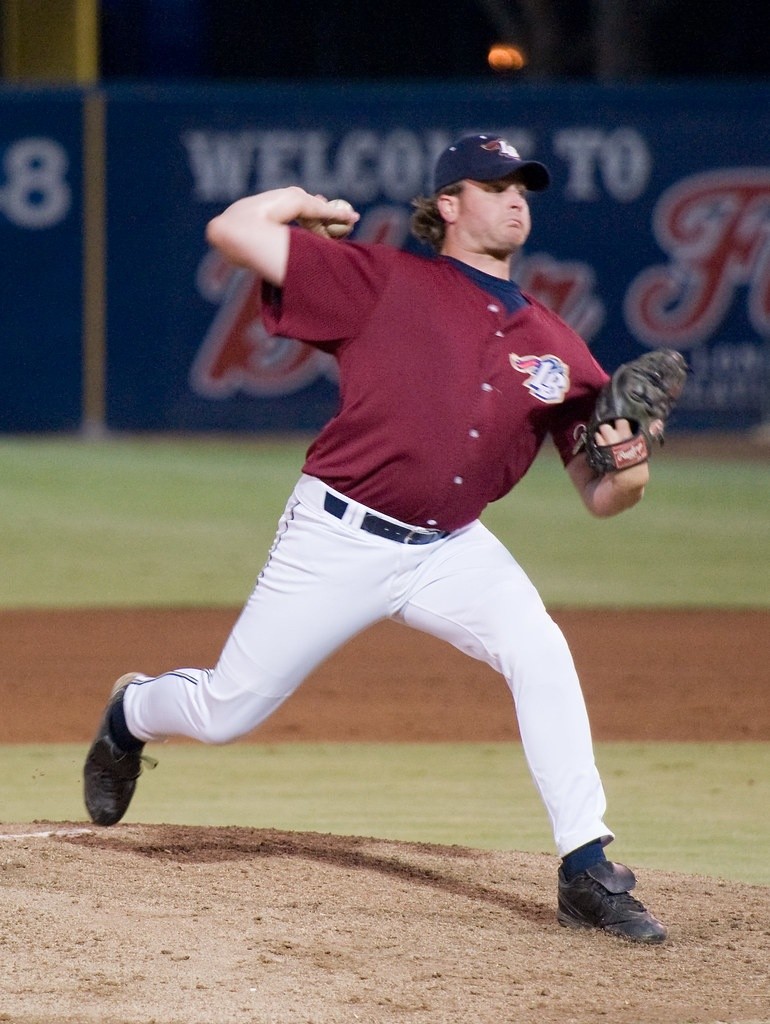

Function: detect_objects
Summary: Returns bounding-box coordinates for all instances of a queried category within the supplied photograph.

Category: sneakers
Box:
[84,672,159,826]
[557,861,668,944]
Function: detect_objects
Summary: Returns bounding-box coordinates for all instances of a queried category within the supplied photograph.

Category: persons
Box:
[84,133,670,943]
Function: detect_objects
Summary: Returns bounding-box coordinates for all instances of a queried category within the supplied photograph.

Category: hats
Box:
[434,134,550,192]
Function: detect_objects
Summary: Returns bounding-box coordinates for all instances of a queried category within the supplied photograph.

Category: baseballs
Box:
[323,199,354,237]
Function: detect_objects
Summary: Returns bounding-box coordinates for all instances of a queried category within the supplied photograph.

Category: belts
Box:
[324,492,451,544]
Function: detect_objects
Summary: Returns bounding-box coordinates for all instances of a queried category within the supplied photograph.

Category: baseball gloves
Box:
[581,347,688,472]
